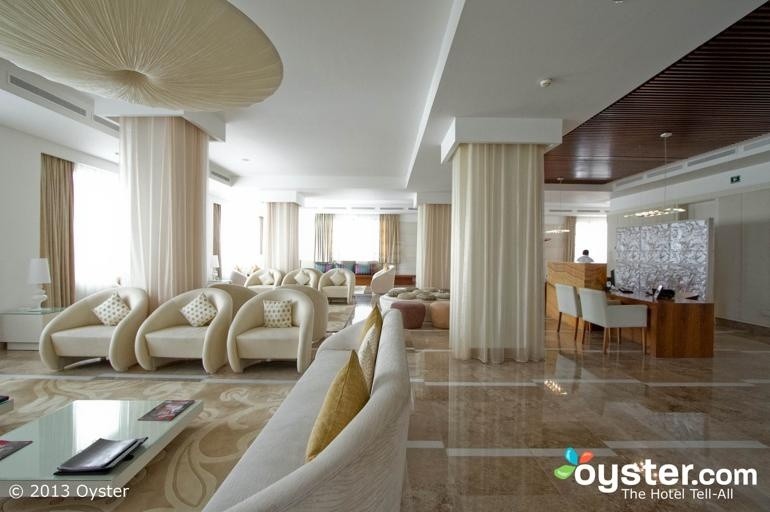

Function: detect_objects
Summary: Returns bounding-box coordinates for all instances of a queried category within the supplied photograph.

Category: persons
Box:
[577,250,594,263]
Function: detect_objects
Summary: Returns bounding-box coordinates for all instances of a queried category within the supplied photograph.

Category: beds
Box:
[0,397,205,512]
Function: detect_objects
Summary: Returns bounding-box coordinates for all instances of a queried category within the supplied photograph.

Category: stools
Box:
[429,301,451,328]
[391,302,426,328]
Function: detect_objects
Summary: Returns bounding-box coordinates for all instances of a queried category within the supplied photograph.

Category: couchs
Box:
[203,309,412,512]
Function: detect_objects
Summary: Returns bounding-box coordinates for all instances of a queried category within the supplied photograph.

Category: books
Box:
[57,438,141,471]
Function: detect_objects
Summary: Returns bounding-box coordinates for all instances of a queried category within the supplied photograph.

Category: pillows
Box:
[305,304,382,462]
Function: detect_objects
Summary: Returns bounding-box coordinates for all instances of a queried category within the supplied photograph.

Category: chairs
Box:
[554,284,621,341]
[577,287,647,356]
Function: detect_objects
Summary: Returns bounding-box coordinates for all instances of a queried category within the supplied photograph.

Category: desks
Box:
[0,308,61,351]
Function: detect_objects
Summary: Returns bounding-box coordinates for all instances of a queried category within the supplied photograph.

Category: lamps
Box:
[209,254,222,279]
[25,257,51,311]
[543,177,570,236]
[633,132,688,217]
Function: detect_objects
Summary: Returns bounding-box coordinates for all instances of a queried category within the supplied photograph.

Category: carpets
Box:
[0,365,302,512]
